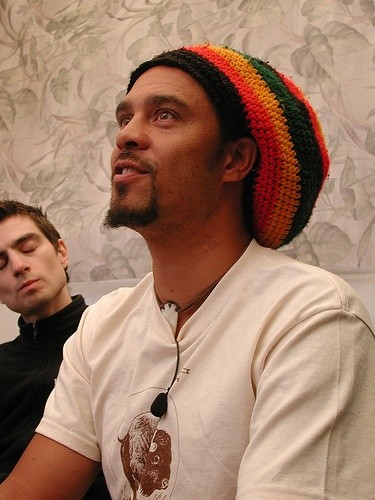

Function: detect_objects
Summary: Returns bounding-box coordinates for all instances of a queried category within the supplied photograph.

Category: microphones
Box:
[151,340,180,416]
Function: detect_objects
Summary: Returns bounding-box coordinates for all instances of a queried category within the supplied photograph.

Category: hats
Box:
[126,42,330,250]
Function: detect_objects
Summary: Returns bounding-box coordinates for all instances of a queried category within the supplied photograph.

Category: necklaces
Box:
[153,272,225,339]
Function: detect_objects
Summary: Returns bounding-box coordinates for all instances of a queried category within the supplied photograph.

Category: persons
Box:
[0,199,113,500]
[0,44,375,500]
